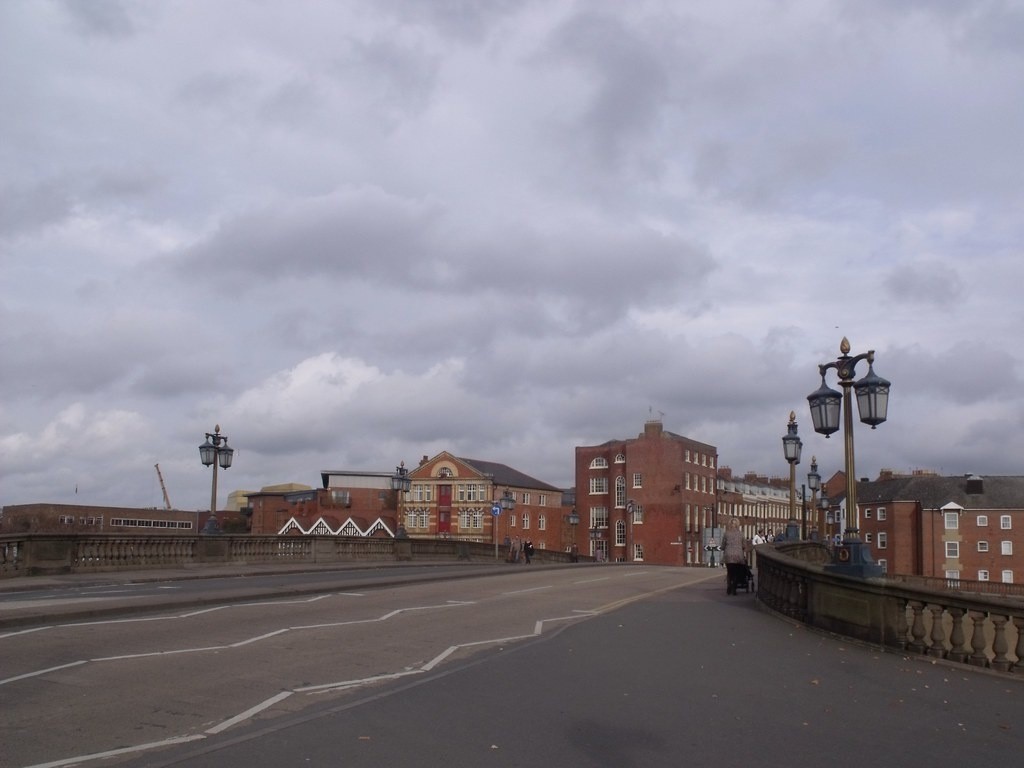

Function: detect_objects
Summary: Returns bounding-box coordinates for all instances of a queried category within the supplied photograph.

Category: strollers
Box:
[733,553,754,594]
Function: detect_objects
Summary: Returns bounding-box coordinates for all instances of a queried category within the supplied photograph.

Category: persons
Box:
[522,537,532,565]
[773,529,785,542]
[509,534,522,563]
[718,518,747,596]
[571,542,578,563]
[594,546,602,562]
[765,529,774,542]
[752,528,767,545]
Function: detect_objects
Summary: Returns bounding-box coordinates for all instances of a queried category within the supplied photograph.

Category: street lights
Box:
[392,460,412,539]
[499,485,516,544]
[808,456,821,540]
[806,336,891,579]
[198,424,234,534]
[782,410,803,541]
[819,482,830,540]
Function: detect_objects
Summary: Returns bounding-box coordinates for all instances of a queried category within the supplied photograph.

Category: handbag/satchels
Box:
[529,549,534,556]
[520,552,525,559]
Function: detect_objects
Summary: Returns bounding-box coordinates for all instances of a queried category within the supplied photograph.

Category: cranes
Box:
[154,463,172,510]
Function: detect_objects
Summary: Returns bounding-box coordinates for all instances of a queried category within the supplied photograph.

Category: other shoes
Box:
[726,584,737,594]
